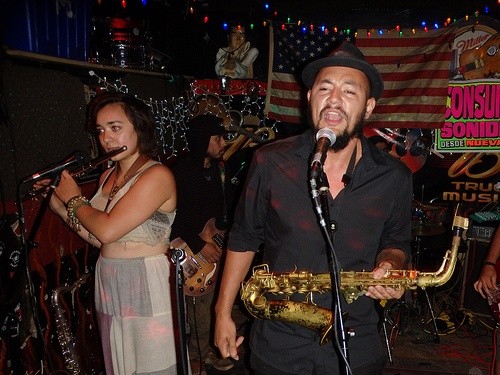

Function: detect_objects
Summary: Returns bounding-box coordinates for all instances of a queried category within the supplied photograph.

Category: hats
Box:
[187,115,224,156]
[302,43,385,101]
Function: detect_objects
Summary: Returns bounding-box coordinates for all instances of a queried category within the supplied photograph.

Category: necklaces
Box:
[103,173,126,212]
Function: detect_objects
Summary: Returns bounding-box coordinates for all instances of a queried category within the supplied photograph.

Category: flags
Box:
[266,31,455,129]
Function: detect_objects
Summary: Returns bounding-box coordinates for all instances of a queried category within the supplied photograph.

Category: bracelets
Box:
[482,261,497,267]
[66,195,91,232]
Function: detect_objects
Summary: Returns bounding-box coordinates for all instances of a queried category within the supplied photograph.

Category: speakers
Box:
[460,237,500,317]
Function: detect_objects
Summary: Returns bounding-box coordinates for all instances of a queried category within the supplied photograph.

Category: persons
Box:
[214,45,412,375]
[166,115,234,375]
[474,225,500,300]
[367,135,390,153]
[32,93,177,375]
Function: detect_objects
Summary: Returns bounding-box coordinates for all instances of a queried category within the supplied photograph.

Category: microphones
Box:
[310,127,336,181]
[23,152,86,182]
[171,249,188,265]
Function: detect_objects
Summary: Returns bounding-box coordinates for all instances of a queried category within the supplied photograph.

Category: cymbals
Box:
[411,222,446,236]
[411,203,439,211]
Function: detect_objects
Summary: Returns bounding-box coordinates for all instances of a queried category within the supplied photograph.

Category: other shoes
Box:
[207,355,234,371]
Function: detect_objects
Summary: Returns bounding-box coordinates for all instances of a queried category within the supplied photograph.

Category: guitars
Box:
[170,217,228,296]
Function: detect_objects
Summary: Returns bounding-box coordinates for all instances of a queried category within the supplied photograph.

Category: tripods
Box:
[388,216,440,348]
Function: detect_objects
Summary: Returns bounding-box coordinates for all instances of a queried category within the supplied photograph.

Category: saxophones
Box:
[239,216,469,346]
[50,275,81,375]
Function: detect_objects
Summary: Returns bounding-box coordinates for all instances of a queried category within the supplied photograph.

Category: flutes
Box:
[14,145,128,207]
